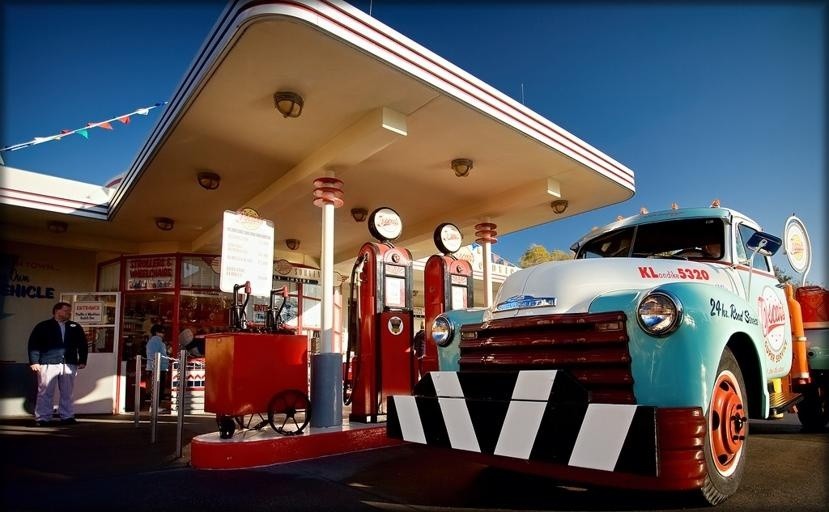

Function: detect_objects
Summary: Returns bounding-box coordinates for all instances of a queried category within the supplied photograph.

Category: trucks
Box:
[386,197,829,506]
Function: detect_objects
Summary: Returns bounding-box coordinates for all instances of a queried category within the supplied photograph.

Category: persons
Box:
[27,302,88,427]
[145,324,173,414]
[179,327,205,387]
[696,224,720,257]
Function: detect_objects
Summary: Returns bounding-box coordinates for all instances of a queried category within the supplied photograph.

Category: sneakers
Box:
[61,418,79,425]
[34,420,50,427]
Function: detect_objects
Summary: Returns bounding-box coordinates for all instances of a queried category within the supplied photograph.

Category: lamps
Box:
[195,169,221,193]
[271,88,305,122]
[284,237,304,252]
[153,215,176,233]
[549,198,570,216]
[449,157,476,179]
[351,207,369,223]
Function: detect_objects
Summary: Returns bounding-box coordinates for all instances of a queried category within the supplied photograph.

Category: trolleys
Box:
[195,281,312,440]
